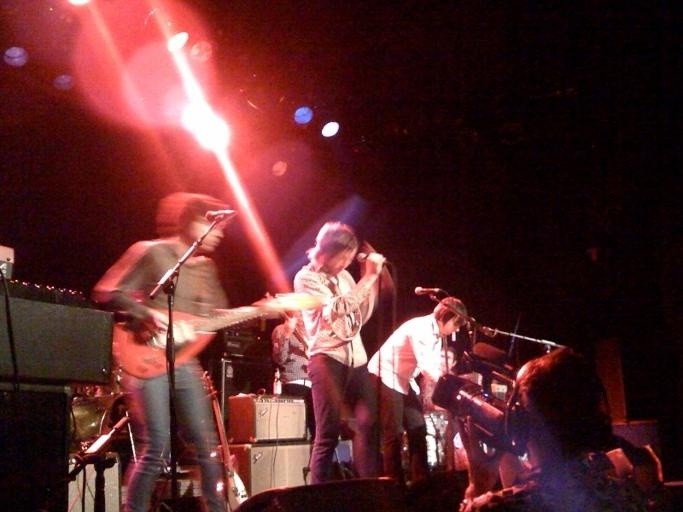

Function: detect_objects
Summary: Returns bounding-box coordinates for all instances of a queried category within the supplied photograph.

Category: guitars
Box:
[112,292,323,378]
[265,293,311,357]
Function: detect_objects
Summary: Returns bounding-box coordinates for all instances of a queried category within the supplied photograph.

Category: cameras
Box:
[432,374,530,457]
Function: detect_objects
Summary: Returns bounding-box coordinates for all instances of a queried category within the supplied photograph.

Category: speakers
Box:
[1,384,70,512]
[229,393,307,441]
[218,445,311,498]
[206,359,280,437]
[2,274,112,384]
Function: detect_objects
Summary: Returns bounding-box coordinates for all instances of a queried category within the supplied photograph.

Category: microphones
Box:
[356,252,389,264]
[414,287,440,295]
[204,209,235,222]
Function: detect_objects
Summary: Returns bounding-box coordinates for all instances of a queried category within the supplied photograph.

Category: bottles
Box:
[273,367,282,399]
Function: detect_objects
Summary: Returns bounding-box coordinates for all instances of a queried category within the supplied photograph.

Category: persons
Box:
[90,191,274,512]
[606,446,683,512]
[367,297,468,487]
[455,346,648,512]
[293,220,389,486]
[272,312,317,450]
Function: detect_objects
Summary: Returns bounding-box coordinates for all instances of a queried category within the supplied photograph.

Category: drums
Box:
[401,411,448,473]
[69,384,134,463]
